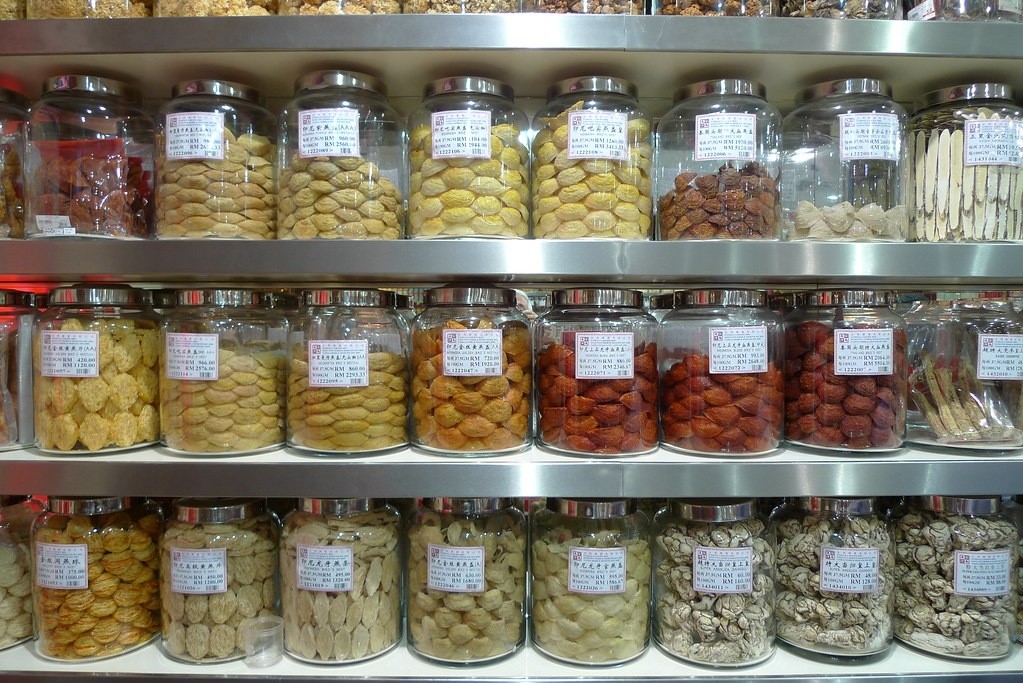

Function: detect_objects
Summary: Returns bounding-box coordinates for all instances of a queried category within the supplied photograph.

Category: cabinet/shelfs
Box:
[0,10,1023,683]
